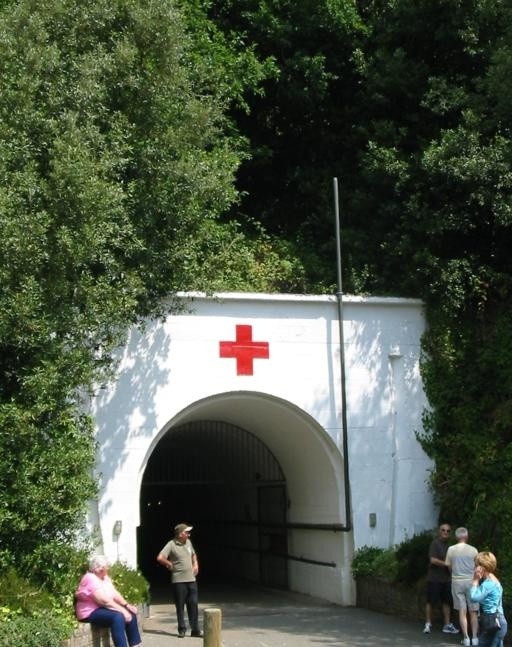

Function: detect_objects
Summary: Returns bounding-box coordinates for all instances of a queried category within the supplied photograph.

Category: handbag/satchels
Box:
[480,613,501,634]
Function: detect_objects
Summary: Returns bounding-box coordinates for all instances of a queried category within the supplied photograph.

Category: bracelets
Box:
[124,603,128,607]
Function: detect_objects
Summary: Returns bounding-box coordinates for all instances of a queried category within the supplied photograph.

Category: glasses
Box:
[442,529,450,532]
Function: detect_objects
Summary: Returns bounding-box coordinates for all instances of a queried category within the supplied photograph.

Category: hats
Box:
[174,523,193,533]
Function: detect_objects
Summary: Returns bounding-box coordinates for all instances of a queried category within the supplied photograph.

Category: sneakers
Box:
[442,623,460,633]
[191,631,203,637]
[423,623,432,633]
[461,639,470,645]
[472,638,479,645]
[178,632,185,638]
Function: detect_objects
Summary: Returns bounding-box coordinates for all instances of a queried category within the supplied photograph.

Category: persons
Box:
[75,556,142,645]
[468,551,507,647]
[424,524,461,634]
[445,527,479,646]
[158,524,201,637]
